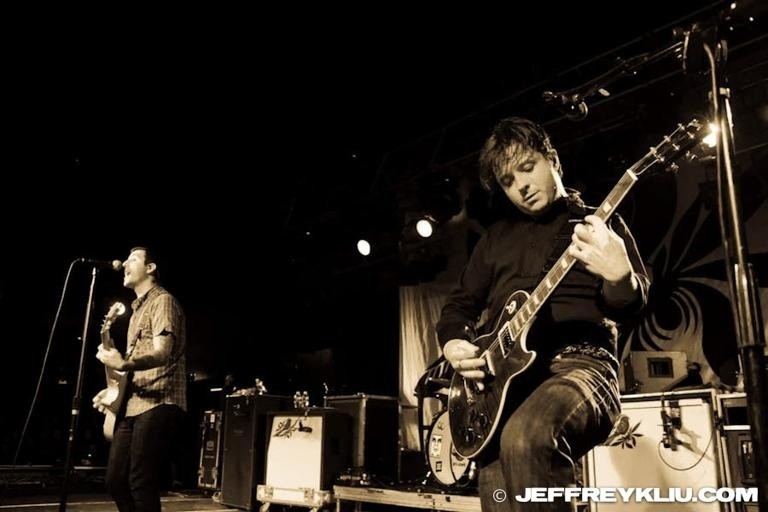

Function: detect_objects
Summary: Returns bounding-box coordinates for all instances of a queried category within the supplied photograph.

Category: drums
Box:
[426,408,480,492]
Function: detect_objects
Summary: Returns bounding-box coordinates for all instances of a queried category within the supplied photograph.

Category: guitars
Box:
[448,112,711,461]
[100,301,127,440]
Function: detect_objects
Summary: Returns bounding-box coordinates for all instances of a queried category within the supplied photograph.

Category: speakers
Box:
[323,394,399,485]
[220,394,295,510]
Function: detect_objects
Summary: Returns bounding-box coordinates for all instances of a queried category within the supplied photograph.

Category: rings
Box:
[458,359,463,369]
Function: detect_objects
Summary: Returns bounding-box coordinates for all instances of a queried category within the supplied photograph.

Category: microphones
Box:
[80,257,122,271]
[543,91,589,121]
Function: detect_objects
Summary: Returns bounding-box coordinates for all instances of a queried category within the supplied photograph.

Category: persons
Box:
[435,112,651,512]
[92,246,188,512]
[666,362,703,391]
